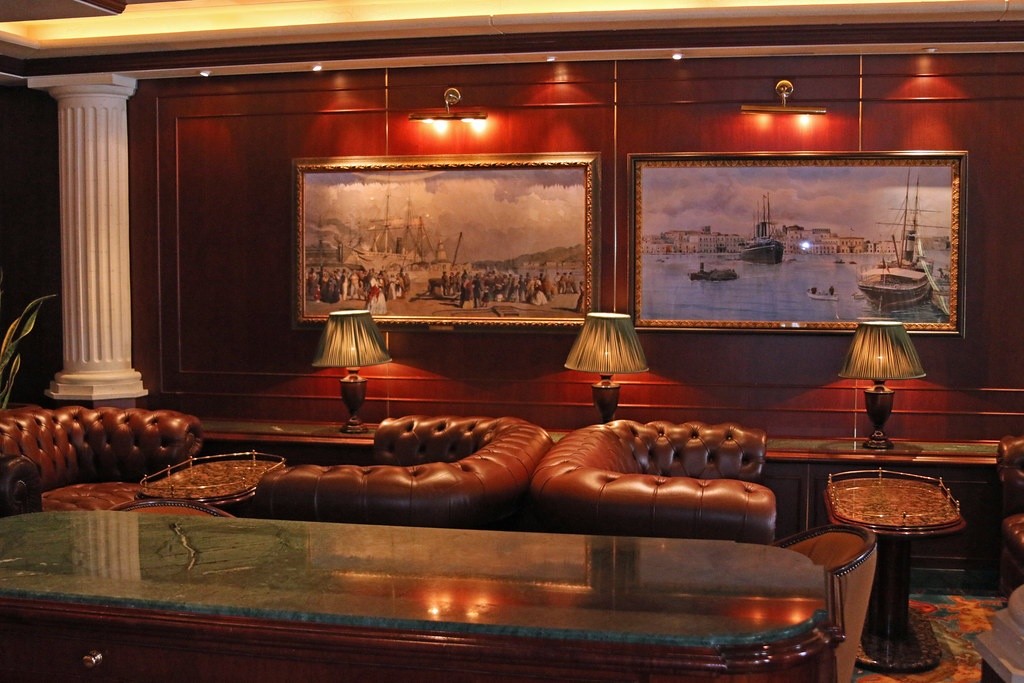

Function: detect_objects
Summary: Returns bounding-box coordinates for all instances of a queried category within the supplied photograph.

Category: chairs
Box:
[108,497,234,518]
[772,524,877,683]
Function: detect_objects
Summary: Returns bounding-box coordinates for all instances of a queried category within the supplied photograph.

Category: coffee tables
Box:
[140,450,285,518]
[823,468,966,672]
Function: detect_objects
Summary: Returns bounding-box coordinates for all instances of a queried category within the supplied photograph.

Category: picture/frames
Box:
[291,152,600,335]
[627,150,969,340]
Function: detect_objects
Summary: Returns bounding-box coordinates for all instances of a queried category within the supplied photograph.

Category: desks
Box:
[199,419,1004,594]
[0,511,839,683]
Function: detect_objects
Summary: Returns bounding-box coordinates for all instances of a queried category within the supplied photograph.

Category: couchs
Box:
[998,436,1024,587]
[0,406,202,518]
[256,413,555,533]
[531,417,776,546]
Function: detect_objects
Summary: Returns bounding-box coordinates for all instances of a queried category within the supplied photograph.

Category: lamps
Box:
[408,87,488,121]
[311,310,391,434]
[836,321,925,449]
[564,312,648,424]
[739,80,826,115]
